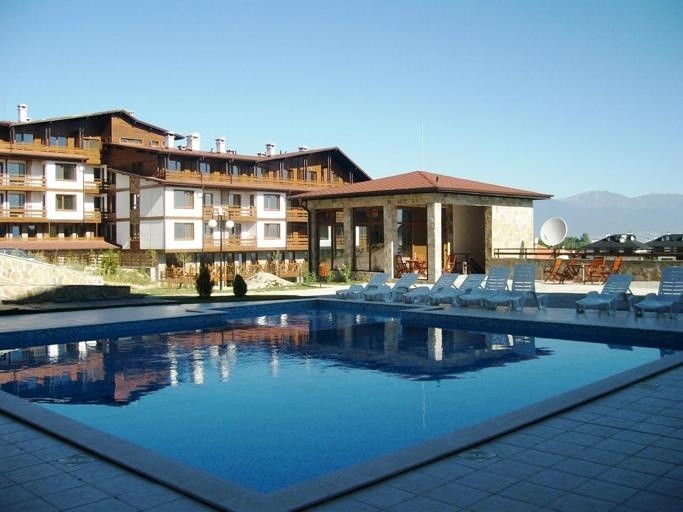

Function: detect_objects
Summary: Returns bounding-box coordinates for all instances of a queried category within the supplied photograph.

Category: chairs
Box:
[336,254,547,315]
[575,266,683,320]
[543,255,622,286]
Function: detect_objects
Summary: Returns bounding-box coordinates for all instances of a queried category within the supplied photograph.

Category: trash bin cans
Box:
[319,261,329,277]
[462,261,468,275]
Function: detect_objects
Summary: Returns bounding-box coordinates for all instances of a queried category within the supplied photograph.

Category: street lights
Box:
[209,208,235,290]
